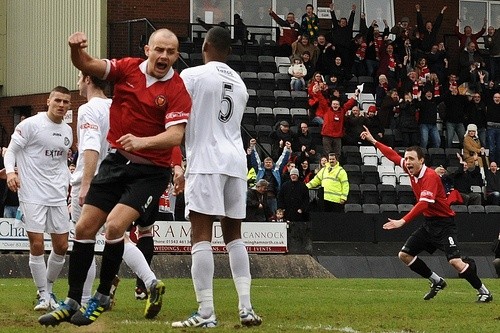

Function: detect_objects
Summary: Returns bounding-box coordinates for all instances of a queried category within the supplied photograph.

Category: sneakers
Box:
[171,310,218,328]
[145,278,166,319]
[36,288,60,311]
[38,300,111,326]
[239,307,263,325]
[108,275,120,309]
[478,292,493,303]
[134,286,147,299]
[423,277,447,300]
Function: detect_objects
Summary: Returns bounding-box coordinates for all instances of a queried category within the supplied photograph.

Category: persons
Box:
[69,66,165,320]
[67,142,79,166]
[158,2,499,244]
[0,168,19,218]
[36,28,192,328]
[171,26,263,327]
[66,163,77,219]
[4,85,73,309]
[360,124,492,302]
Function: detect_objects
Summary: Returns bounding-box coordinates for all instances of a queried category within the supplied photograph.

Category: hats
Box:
[289,168,299,177]
[463,123,479,139]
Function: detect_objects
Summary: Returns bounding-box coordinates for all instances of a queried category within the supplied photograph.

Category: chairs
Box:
[172,26,500,245]
